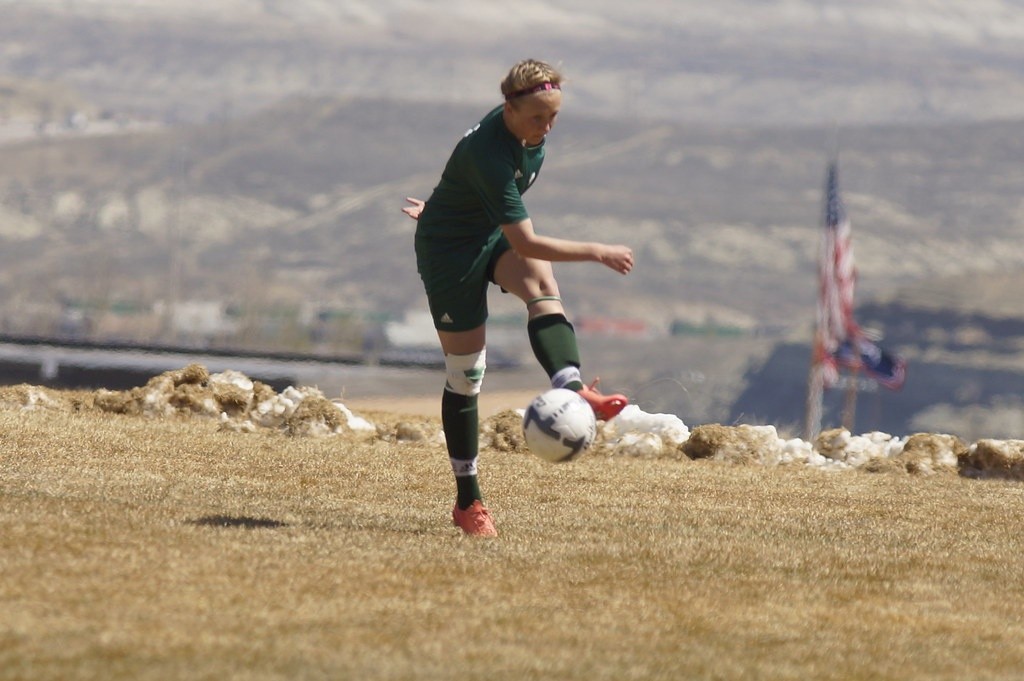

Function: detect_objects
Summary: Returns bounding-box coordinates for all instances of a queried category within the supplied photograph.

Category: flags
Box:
[812,161,905,389]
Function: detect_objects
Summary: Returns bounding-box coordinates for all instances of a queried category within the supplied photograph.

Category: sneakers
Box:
[452,498,498,539]
[577,377,628,422]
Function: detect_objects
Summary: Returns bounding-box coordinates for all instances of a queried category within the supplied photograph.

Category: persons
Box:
[402,59,634,536]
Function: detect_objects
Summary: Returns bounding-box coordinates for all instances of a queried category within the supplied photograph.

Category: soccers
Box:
[521,387,597,464]
